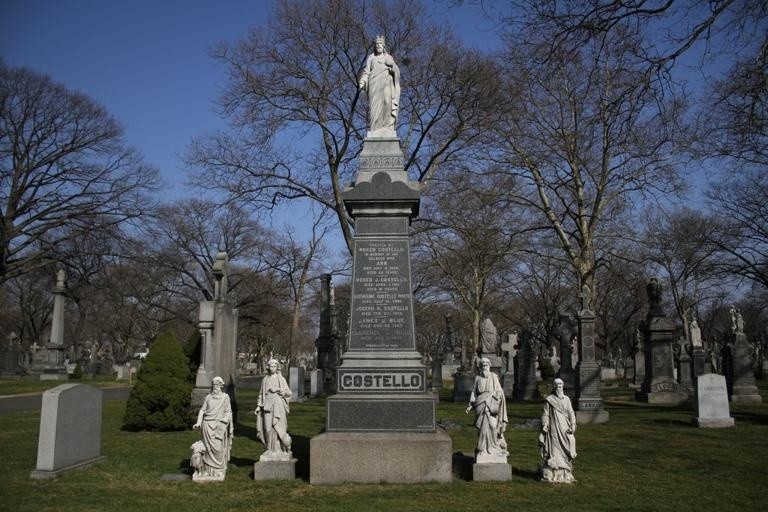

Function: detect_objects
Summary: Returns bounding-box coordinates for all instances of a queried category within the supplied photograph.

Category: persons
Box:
[358,35,402,128]
[252,358,294,453]
[465,357,509,452]
[191,376,234,476]
[730,304,737,329]
[733,307,746,332]
[538,378,578,470]
[689,318,699,328]
